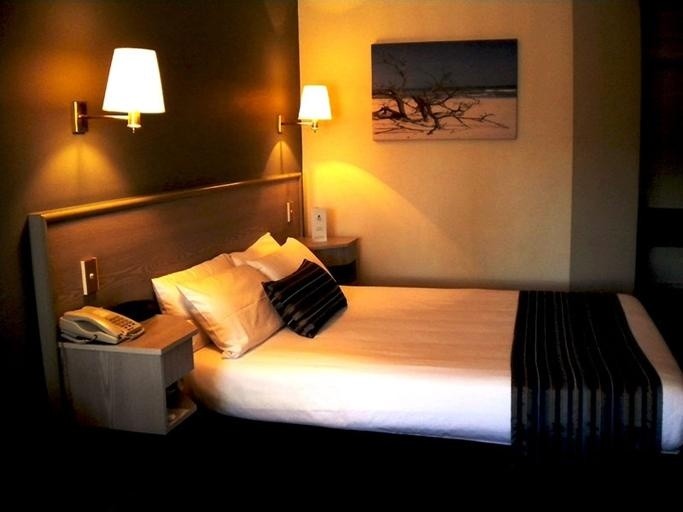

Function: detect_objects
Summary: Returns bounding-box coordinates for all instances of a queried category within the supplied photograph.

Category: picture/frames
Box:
[313,208,327,243]
[372,39,519,142]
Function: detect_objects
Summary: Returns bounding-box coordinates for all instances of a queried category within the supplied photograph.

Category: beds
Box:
[28,172,683,456]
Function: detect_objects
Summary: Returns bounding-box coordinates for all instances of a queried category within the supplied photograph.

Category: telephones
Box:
[59,306,145,344]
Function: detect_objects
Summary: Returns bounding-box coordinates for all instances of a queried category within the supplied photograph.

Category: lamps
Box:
[278,84,332,134]
[73,48,166,135]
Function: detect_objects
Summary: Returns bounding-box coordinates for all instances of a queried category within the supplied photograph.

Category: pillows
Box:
[151,252,235,353]
[247,238,337,281]
[176,265,285,360]
[230,232,281,267]
[261,259,348,338]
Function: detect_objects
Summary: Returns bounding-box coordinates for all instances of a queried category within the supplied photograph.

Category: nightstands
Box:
[57,313,198,437]
[298,235,359,283]
